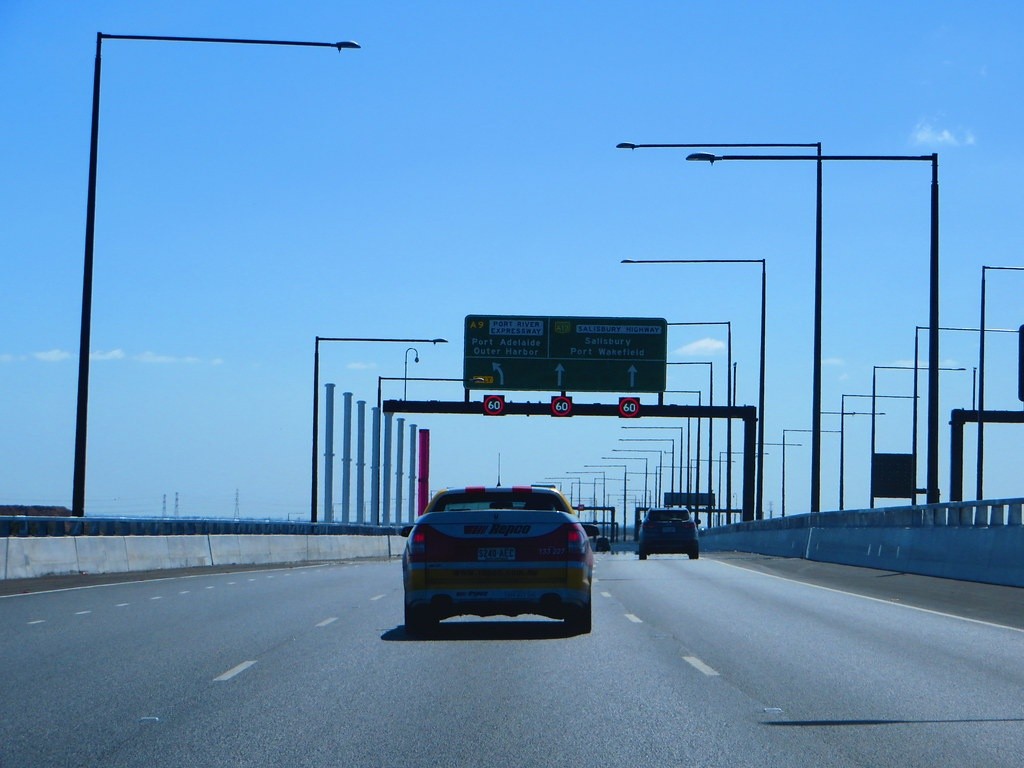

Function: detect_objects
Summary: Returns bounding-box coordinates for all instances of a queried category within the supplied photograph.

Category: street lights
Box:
[782,428,840,516]
[622,426,684,507]
[690,458,736,518]
[621,259,766,521]
[684,152,940,504]
[71,29,363,509]
[377,375,484,525]
[869,366,967,508]
[654,465,694,508]
[719,452,770,524]
[612,449,662,509]
[839,393,920,511]
[536,456,648,542]
[616,142,819,515]
[312,335,448,522]
[619,438,675,509]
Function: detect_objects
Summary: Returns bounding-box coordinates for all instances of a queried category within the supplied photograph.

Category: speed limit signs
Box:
[618,397,641,418]
[482,394,506,417]
[551,396,574,417]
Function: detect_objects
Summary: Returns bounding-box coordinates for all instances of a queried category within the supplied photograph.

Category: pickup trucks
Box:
[397,482,599,636]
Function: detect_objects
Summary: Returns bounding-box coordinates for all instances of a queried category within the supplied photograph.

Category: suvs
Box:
[638,506,701,561]
